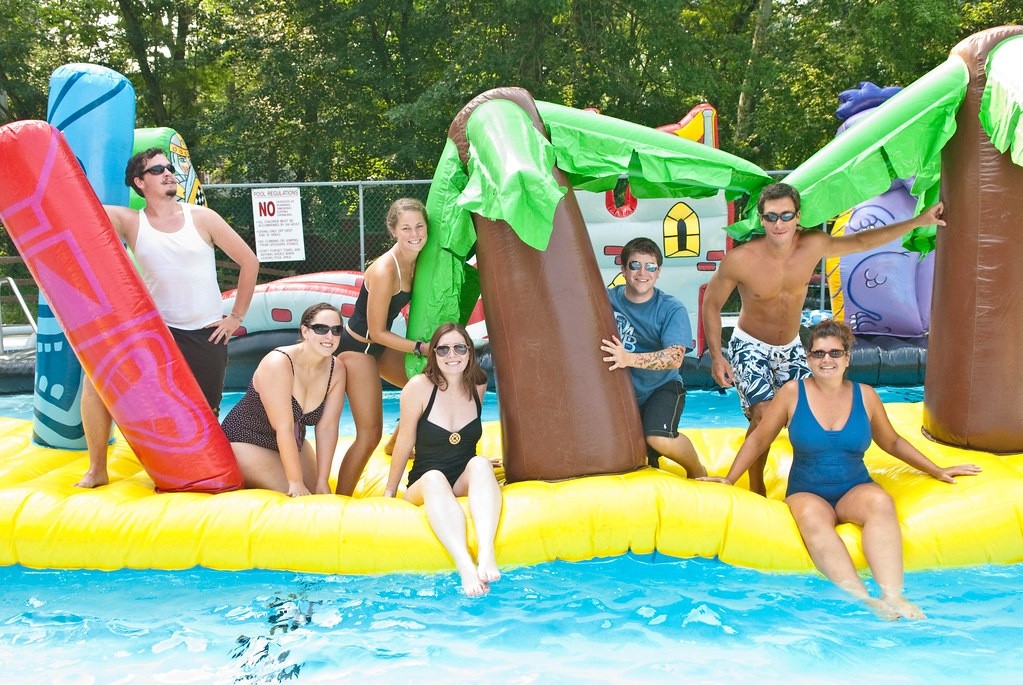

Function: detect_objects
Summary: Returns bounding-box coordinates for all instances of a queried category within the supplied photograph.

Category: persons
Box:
[703,182,948,497]
[384,321,503,598]
[604,237,708,480]
[221,302,347,499]
[693,320,982,600]
[76,146,260,488]
[335,197,431,501]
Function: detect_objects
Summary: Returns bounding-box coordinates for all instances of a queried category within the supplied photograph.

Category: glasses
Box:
[628,261,660,272]
[434,343,470,358]
[305,324,343,336]
[809,349,848,358]
[761,212,798,222]
[138,164,176,179]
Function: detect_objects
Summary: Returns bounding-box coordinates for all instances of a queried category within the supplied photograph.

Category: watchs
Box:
[413,342,422,357]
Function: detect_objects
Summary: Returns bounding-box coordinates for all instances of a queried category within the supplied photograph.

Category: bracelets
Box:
[231,313,243,323]
